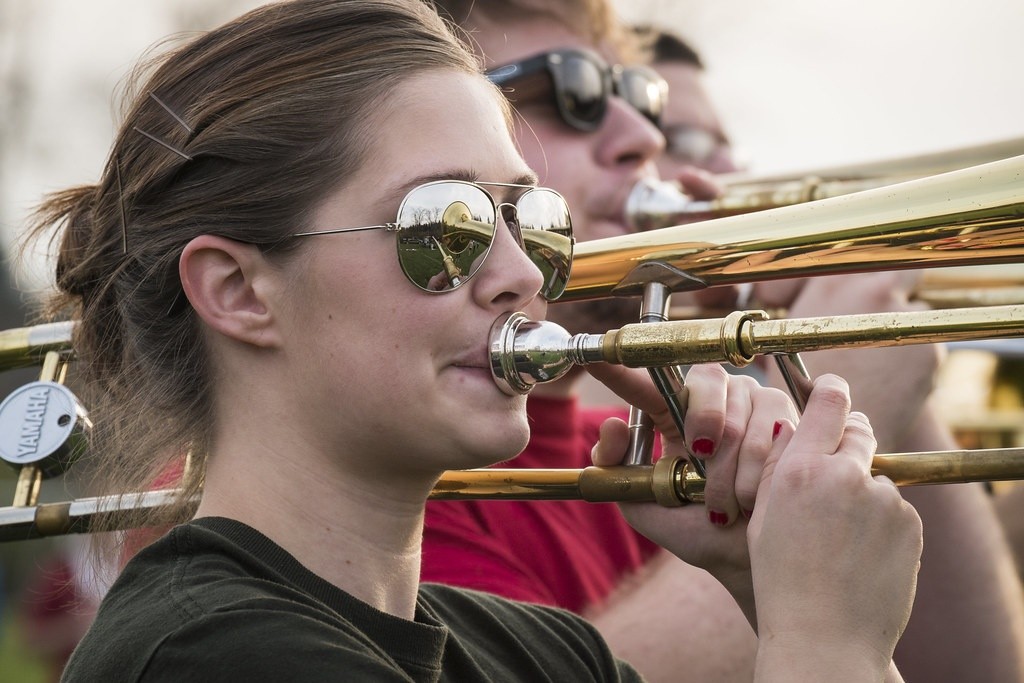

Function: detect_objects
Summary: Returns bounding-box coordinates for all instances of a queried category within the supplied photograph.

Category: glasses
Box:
[278,179,577,301]
[664,123,730,164]
[480,47,669,132]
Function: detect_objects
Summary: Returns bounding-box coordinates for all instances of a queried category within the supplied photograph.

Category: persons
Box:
[35,0,1024,683]
[53,0,921,683]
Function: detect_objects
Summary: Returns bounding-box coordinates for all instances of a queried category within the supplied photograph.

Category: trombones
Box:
[0,156,1024,538]
[621,136,1024,319]
